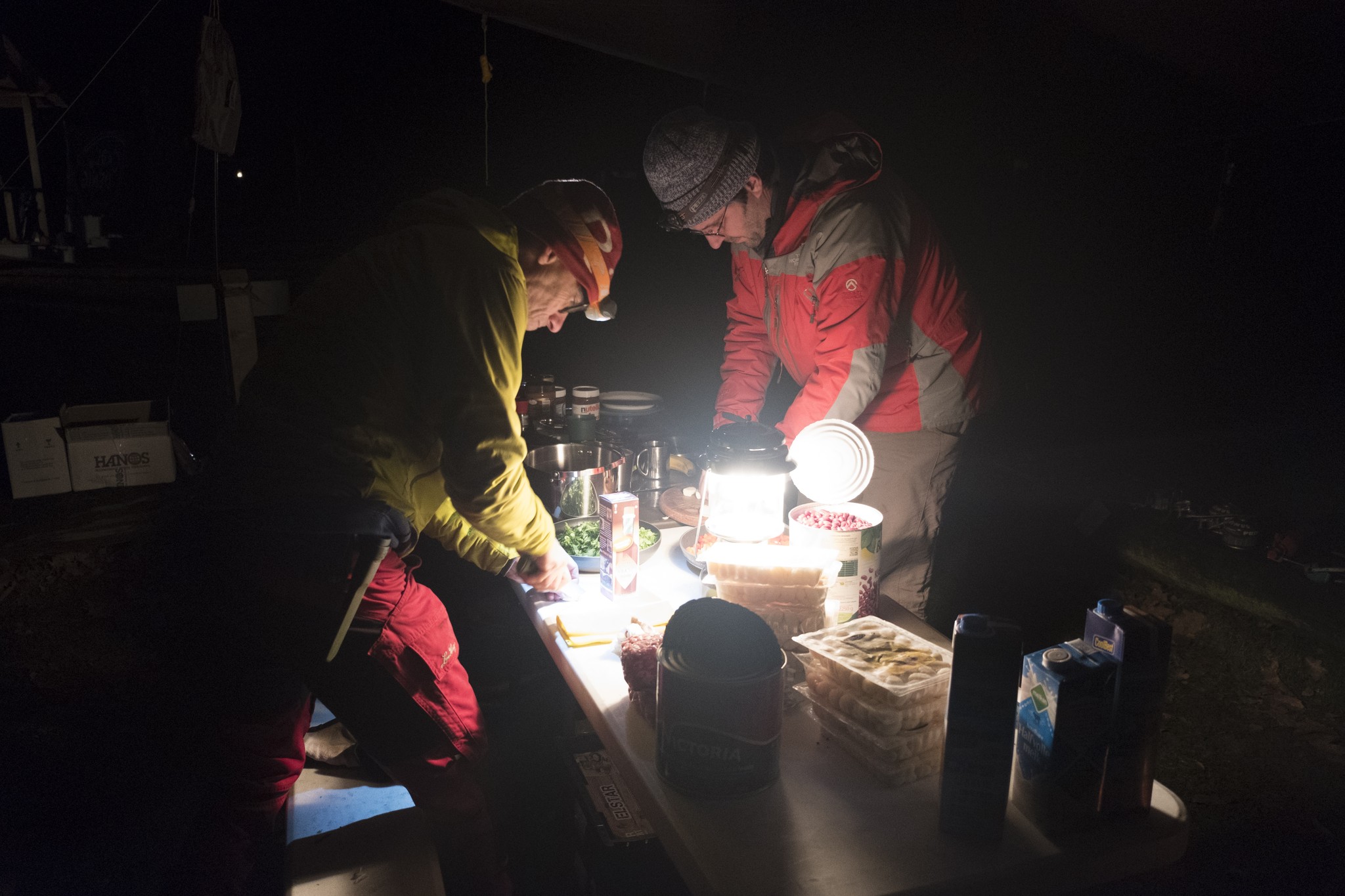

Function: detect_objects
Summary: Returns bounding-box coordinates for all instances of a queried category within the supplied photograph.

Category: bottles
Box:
[529,374,556,428]
[515,381,529,432]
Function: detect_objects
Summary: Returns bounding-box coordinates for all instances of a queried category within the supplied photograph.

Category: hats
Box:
[643,108,761,230]
[493,180,623,323]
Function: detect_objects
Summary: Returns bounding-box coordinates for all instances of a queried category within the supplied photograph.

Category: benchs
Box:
[287,760,445,896]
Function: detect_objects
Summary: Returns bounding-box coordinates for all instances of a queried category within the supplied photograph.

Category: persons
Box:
[643,104,993,625]
[174,178,625,895]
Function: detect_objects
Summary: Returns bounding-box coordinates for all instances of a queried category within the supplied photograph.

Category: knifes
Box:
[516,557,592,608]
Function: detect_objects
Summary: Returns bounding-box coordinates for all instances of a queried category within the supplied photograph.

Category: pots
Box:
[523,443,626,523]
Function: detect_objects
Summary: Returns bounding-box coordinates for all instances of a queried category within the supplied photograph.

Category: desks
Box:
[511,525,1191,896]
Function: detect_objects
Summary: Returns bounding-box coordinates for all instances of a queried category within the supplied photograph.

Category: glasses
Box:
[690,204,725,238]
[556,286,589,314]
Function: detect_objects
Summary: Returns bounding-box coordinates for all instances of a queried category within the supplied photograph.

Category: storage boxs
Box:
[599,491,639,601]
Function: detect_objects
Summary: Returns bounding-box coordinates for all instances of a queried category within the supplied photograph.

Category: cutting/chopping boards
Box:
[659,483,708,528]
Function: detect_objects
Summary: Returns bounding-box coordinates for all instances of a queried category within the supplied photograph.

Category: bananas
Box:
[669,454,695,477]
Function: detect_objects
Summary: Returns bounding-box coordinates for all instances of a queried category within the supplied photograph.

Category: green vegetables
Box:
[555,519,658,557]
[562,478,596,516]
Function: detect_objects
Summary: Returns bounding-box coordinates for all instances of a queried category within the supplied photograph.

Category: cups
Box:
[567,414,596,442]
[635,440,671,479]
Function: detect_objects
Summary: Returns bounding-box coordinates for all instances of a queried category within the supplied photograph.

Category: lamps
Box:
[693,416,796,557]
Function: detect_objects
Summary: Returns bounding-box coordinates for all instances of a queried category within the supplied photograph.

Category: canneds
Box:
[786,418,884,625]
[654,598,786,800]
[515,375,600,431]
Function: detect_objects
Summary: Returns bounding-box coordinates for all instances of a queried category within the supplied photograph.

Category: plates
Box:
[599,390,662,410]
[679,525,790,572]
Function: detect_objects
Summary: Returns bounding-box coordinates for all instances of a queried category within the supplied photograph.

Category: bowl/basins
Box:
[696,541,843,651]
[791,614,954,787]
[553,516,661,572]
